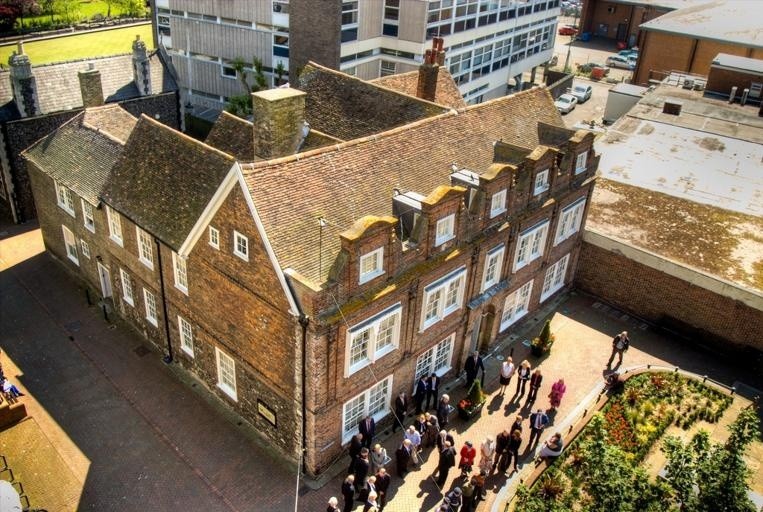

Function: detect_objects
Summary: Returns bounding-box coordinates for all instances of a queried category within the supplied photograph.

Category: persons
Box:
[326,349,566,512]
[605,330,630,366]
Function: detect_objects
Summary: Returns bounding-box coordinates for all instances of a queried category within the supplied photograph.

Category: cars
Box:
[557,26,577,36]
[572,83,592,104]
[604,41,640,70]
[576,61,610,73]
[560,0,584,16]
[566,24,579,29]
[552,93,577,114]
[539,54,558,67]
